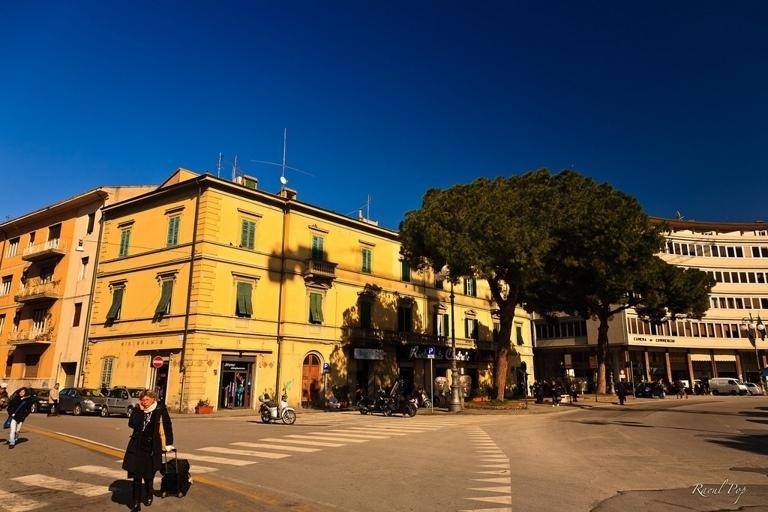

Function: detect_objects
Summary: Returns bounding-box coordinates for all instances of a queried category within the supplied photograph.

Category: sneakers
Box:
[5,438,17,449]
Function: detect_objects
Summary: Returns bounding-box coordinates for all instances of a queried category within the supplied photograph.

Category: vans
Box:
[708,376,748,396]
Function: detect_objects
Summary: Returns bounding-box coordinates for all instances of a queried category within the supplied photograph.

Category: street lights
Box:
[739,316,766,393]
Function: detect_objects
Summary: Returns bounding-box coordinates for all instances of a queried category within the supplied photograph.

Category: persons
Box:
[98,383,141,398]
[121,388,175,511]
[7,386,31,448]
[309,377,411,411]
[47,383,60,417]
[0,382,9,412]
[486,374,712,407]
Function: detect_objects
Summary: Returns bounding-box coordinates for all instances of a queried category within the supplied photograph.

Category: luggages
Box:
[157,448,192,498]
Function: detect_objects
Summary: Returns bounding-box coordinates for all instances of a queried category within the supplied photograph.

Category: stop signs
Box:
[152,355,164,368]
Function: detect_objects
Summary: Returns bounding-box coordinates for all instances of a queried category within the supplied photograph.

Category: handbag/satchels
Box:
[3,415,12,429]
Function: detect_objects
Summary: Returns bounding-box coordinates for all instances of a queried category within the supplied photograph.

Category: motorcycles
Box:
[354,384,431,418]
[258,386,297,425]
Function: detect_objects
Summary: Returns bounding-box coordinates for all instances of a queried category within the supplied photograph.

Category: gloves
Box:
[166,444,175,451]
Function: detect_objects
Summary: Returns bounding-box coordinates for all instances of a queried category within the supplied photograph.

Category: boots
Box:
[144,473,153,506]
[130,473,141,511]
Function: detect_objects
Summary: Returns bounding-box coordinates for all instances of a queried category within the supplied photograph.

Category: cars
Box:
[745,382,763,396]
[636,382,665,399]
[10,386,147,416]
